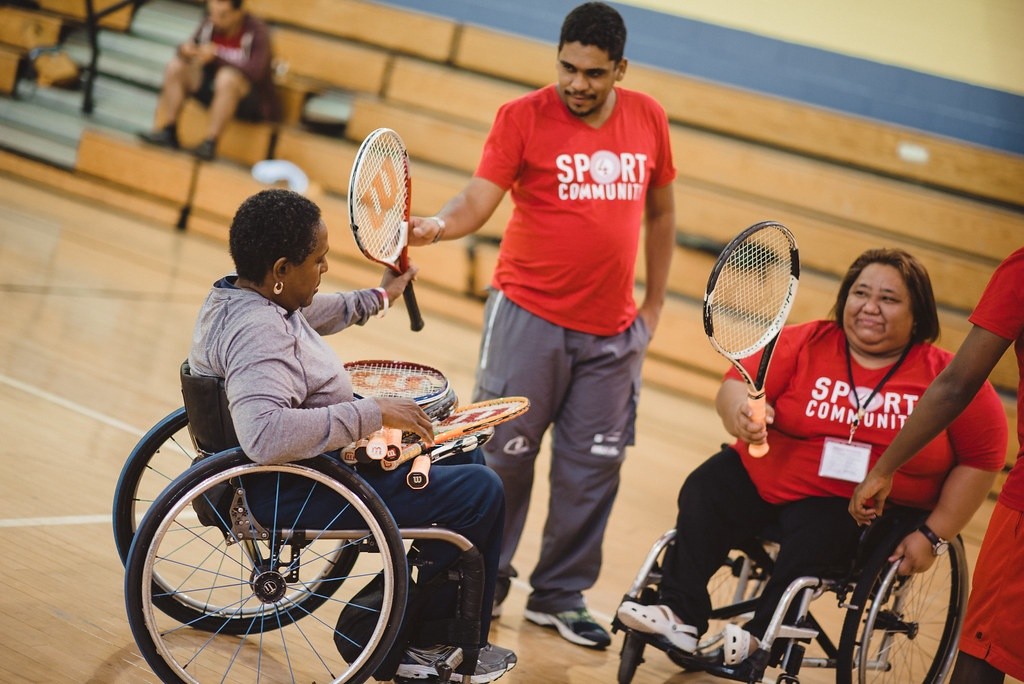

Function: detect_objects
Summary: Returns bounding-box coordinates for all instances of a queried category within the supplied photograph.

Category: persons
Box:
[139,0,279,160]
[187,188,518,684]
[615,248,1009,665]
[393,2,678,651]
[848,246,1024,684]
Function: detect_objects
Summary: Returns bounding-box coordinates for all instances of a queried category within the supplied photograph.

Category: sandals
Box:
[616,600,699,653]
[724,623,761,664]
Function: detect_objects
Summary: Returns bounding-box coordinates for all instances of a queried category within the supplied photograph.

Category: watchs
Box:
[918,521,949,557]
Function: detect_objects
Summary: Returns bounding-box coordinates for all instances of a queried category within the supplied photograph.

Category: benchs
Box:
[0,0,1024,504]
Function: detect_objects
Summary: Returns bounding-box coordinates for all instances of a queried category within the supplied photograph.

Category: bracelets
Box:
[430,216,445,243]
[372,287,389,320]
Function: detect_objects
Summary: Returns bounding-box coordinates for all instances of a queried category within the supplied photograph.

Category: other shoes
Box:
[136,123,178,147]
[188,140,218,162]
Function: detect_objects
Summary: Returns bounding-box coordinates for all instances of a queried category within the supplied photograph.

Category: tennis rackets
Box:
[339,360,531,489]
[350,127,426,332]
[707,223,799,456]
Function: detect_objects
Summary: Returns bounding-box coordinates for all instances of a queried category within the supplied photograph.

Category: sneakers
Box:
[480,642,517,672]
[491,580,511,617]
[524,607,611,647]
[396,640,508,683]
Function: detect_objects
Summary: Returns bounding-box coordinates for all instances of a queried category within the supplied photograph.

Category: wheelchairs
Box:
[108,356,508,684]
[610,508,970,684]
